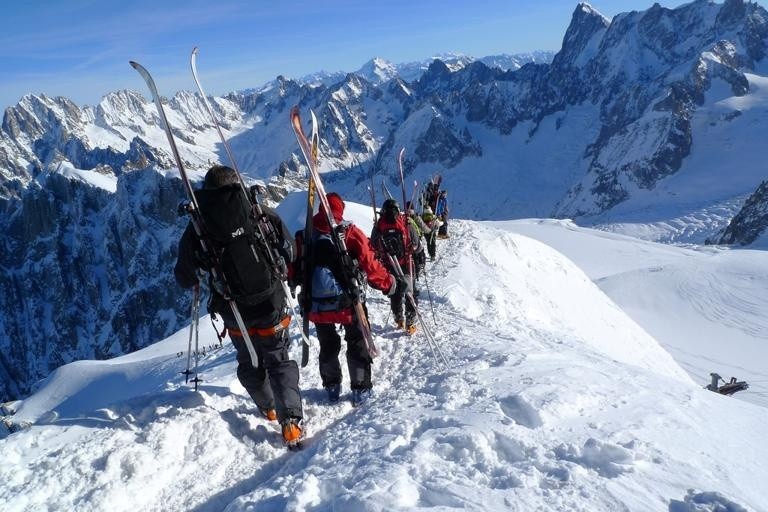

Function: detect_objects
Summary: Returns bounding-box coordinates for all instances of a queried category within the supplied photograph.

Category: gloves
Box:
[397,275,412,297]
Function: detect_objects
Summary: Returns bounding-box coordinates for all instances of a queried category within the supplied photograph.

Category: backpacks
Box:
[374,189,447,268]
[297,220,362,313]
[191,183,288,320]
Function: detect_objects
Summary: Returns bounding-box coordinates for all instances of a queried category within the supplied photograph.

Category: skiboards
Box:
[367,147,442,280]
[289,104,379,366]
[129,47,311,369]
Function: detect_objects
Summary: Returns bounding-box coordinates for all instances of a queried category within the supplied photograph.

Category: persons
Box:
[436,189,451,239]
[404,201,433,241]
[173,165,308,450]
[369,198,425,336]
[285,191,403,407]
[420,204,446,261]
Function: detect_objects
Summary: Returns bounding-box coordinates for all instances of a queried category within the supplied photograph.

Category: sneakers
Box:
[351,389,371,407]
[328,383,342,400]
[259,409,275,420]
[396,319,418,336]
[281,422,303,433]
